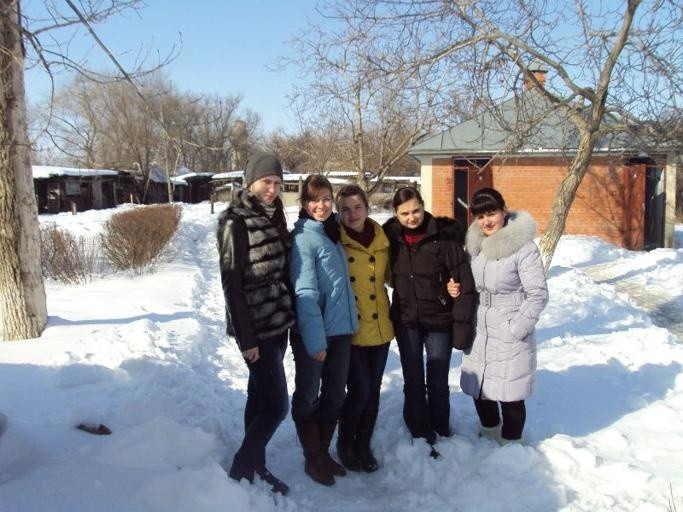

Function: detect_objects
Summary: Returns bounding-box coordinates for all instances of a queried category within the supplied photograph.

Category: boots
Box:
[477,417,525,448]
[319,392,346,477]
[230,439,290,496]
[402,385,438,459]
[426,386,451,437]
[291,394,334,486]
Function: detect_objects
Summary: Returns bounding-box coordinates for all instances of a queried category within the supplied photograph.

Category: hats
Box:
[244,152,284,189]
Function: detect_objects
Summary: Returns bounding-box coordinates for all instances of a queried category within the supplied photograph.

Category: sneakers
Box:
[337,436,377,472]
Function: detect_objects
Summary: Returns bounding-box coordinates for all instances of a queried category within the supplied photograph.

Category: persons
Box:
[380,187,474,459]
[287,172,359,485]
[214,150,299,496]
[445,186,549,446]
[331,186,396,472]
